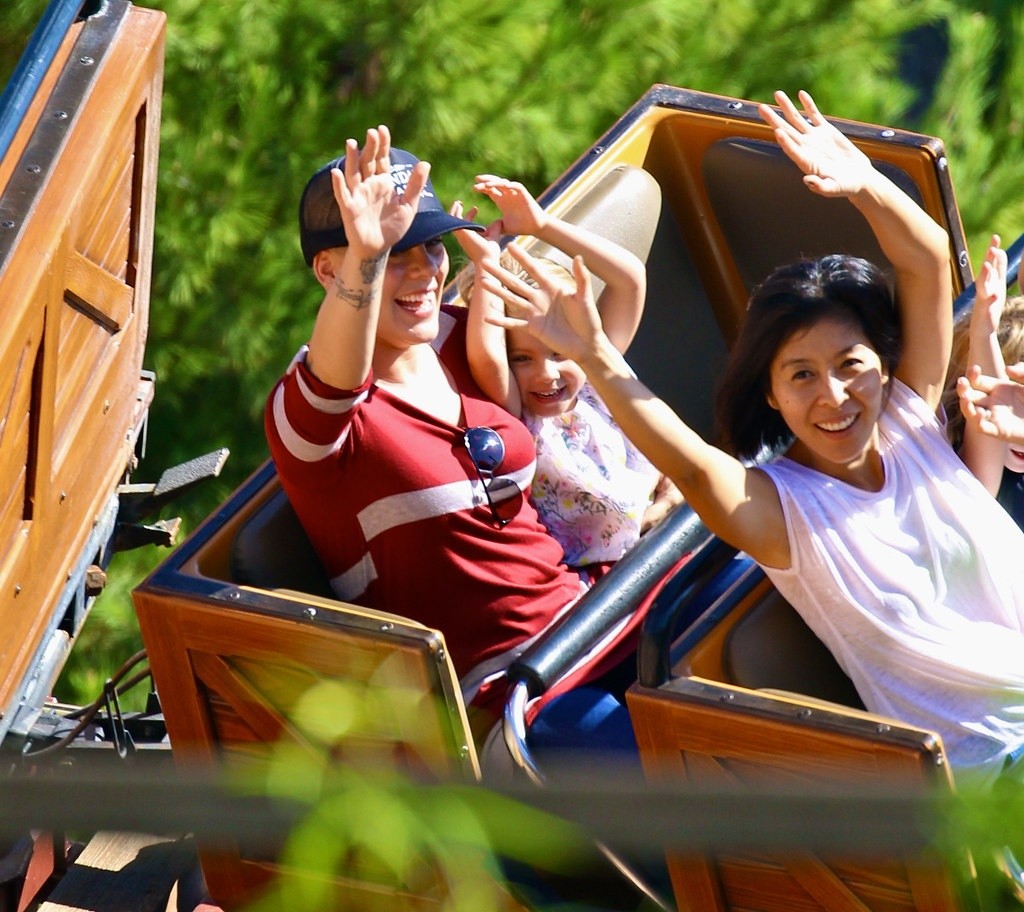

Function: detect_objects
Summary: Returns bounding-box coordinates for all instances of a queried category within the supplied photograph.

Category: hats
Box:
[299,145,486,273]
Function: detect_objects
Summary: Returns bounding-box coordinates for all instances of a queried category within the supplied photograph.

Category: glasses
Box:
[462,426,523,529]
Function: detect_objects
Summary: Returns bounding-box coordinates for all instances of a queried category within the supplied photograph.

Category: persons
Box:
[264,124,684,754]
[450,174,659,568]
[943,234,1024,532]
[481,90,1024,890]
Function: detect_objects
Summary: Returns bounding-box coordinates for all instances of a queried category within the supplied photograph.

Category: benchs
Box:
[0,1,1024,912]
